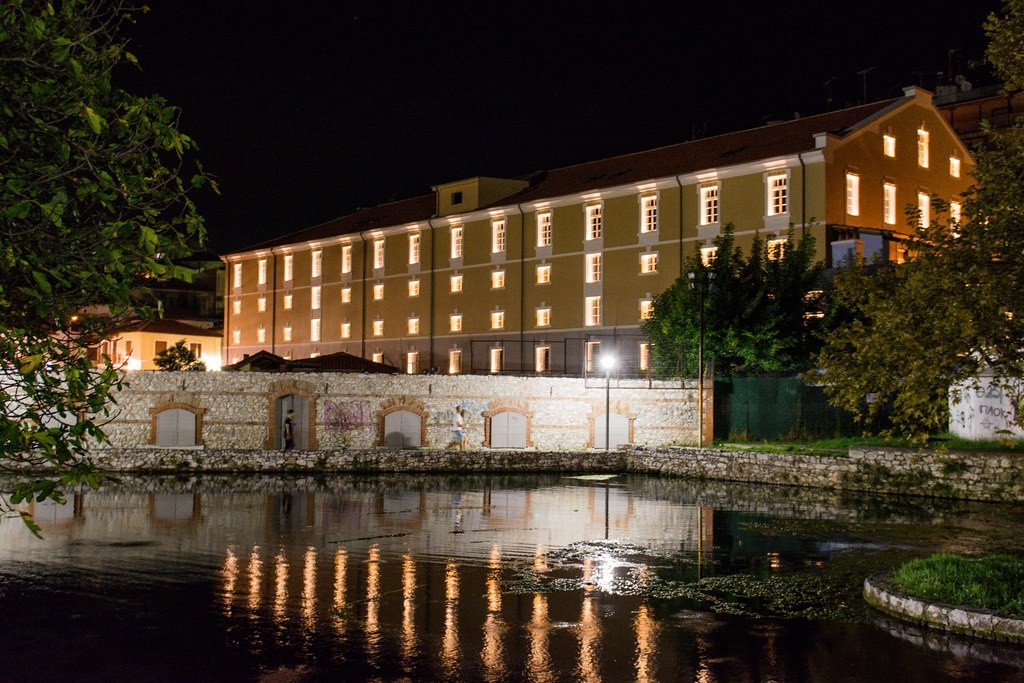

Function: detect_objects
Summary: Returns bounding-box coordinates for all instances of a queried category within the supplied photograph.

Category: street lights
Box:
[600,354,617,451]
[596,482,615,591]
[686,270,717,448]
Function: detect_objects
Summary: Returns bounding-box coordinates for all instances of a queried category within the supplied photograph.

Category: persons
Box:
[284,409,296,450]
[445,406,467,449]
